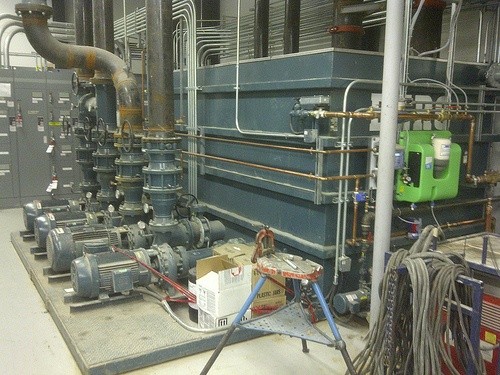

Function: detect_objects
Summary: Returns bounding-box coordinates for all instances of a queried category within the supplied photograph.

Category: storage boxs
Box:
[196,241,286,329]
